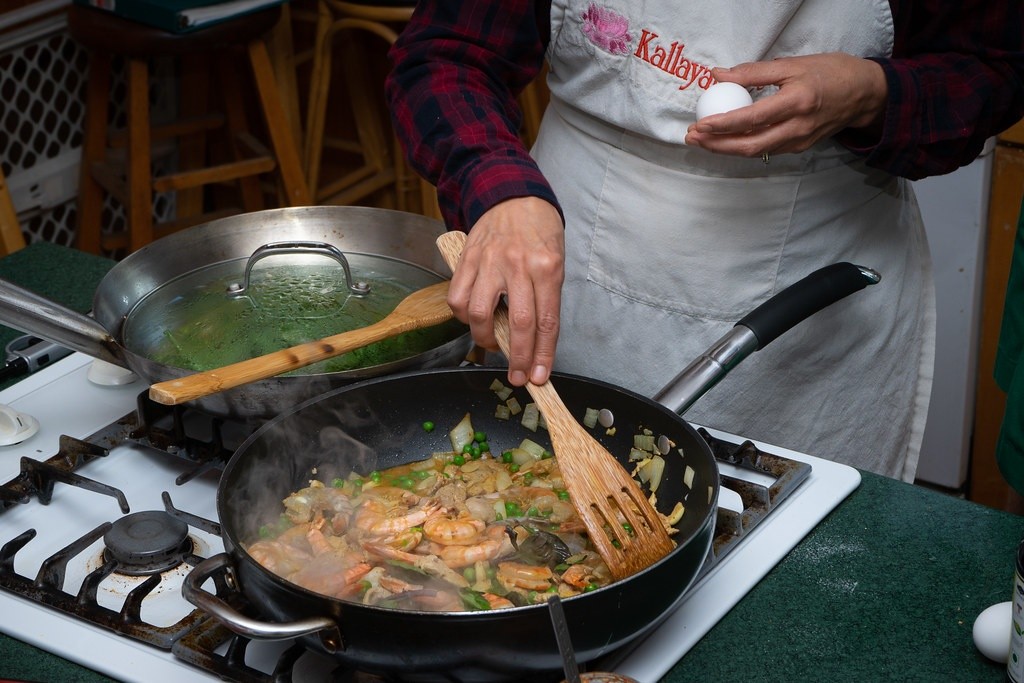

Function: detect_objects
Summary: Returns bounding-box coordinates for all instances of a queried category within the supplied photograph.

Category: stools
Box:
[0,175,26,256]
[181,0,393,227]
[65,4,309,258]
[307,0,543,224]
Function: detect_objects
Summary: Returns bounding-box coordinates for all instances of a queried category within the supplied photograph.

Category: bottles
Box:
[1006,538,1024,683]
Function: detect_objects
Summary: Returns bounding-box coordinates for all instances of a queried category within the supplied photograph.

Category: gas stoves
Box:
[0,347,861,683]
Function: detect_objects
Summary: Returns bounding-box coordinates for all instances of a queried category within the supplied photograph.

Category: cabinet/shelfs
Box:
[965,112,1024,513]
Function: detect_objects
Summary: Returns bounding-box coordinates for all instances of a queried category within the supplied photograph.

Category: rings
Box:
[759,150,771,164]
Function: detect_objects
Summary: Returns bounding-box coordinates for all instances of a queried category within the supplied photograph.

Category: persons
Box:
[372,0,1024,484]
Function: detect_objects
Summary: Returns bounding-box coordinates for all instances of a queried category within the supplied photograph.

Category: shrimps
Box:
[238,456,663,613]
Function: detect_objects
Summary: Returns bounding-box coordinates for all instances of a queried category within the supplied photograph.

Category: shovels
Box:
[148,279,455,405]
[436,230,675,582]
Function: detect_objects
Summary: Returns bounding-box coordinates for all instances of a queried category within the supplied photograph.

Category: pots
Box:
[0,205,473,418]
[181,261,883,677]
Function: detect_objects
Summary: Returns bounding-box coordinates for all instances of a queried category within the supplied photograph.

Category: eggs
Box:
[697,81,753,135]
[973,600,1013,665]
